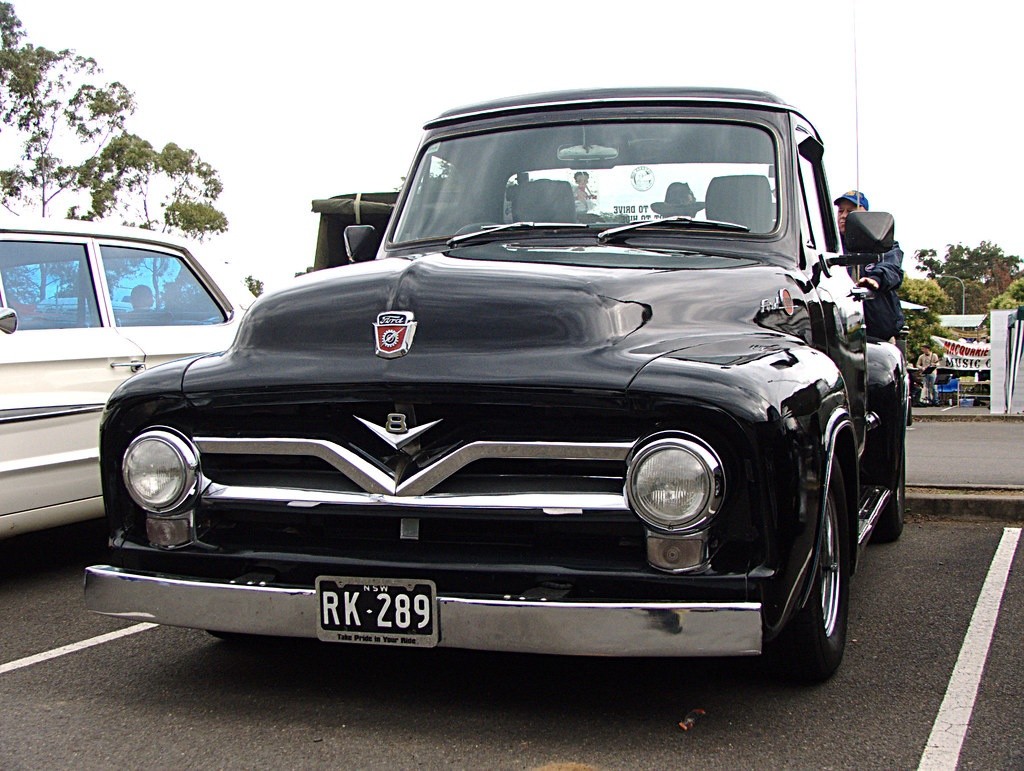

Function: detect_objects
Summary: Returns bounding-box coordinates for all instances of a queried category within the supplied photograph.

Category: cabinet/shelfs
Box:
[957,382,990,408]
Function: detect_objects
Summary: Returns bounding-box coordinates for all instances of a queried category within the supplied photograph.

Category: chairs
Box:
[125,285,156,322]
[512,179,576,224]
[705,175,774,232]
[937,379,961,406]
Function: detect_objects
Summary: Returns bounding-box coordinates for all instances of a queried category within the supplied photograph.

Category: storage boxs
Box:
[960,399,974,407]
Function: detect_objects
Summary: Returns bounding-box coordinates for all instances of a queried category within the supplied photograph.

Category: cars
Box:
[0,218,254,540]
[84,87,909,690]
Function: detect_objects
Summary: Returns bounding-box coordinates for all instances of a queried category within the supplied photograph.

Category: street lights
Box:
[935,274,965,315]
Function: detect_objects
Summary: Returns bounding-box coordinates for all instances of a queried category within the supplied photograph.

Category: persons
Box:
[897,348,922,406]
[650,182,705,222]
[834,190,907,359]
[916,345,941,406]
[122,283,176,326]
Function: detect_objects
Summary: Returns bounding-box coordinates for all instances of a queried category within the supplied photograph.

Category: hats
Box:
[651,182,705,216]
[833,190,869,211]
